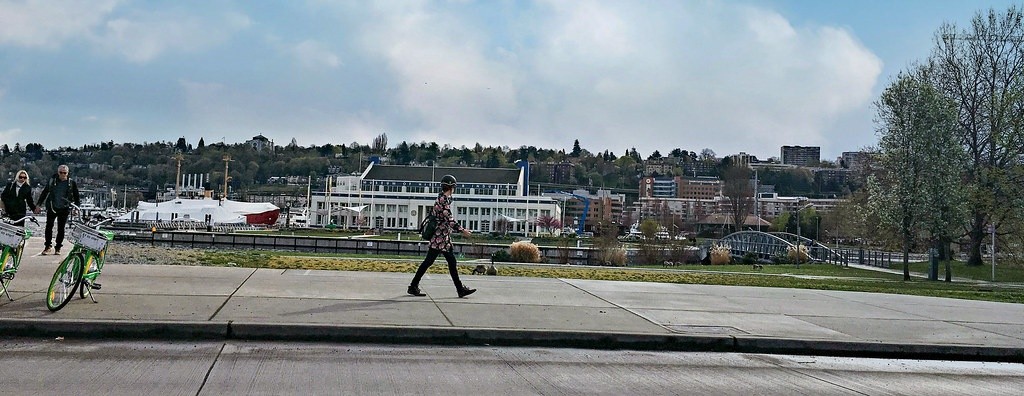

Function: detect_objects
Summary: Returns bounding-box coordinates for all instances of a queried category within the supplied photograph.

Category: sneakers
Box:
[55,249,61,255]
[42,247,51,254]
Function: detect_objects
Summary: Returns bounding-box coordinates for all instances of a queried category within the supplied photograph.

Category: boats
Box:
[48,157,311,228]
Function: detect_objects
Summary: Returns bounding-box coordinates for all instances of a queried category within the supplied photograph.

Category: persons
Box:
[34,163,81,256]
[1,170,40,238]
[406,174,478,299]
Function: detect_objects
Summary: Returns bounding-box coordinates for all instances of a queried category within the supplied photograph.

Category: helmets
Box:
[441,175,457,186]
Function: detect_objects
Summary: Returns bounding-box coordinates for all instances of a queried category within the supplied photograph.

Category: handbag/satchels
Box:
[420,215,437,239]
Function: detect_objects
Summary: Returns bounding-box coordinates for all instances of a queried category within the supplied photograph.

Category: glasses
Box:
[19,176,27,179]
[59,171,68,174]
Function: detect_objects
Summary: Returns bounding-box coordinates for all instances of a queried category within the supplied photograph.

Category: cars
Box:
[565,221,677,240]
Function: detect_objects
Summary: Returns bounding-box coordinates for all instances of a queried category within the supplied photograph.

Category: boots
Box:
[408,286,426,296]
[457,286,476,298]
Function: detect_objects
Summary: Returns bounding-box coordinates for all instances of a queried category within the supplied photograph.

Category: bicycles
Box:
[47,197,116,312]
[0,209,40,301]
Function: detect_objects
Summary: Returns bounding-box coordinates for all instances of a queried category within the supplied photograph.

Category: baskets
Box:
[0,222,25,248]
[67,221,109,254]
